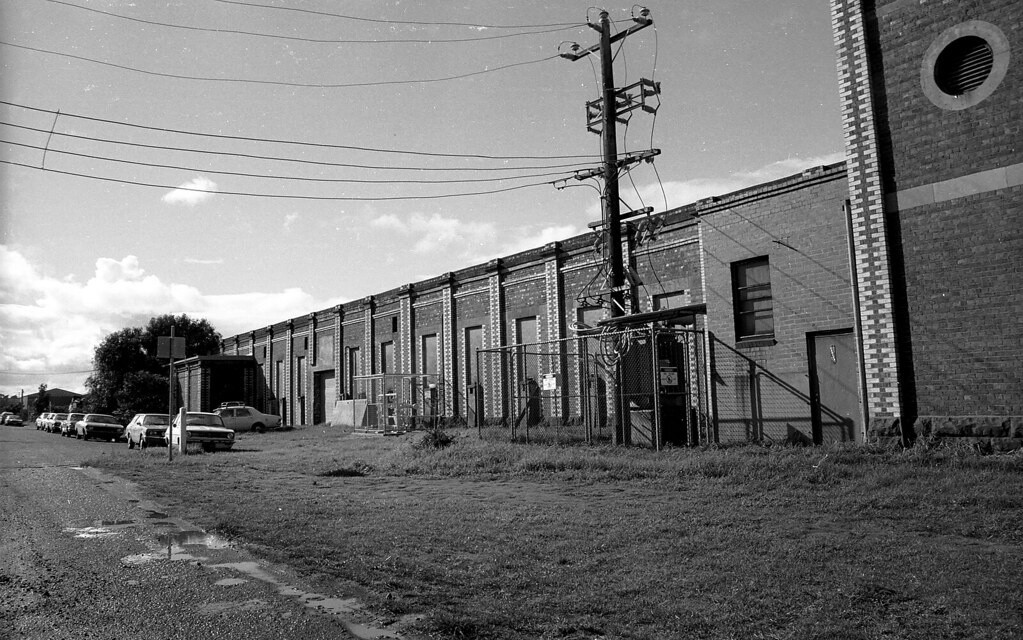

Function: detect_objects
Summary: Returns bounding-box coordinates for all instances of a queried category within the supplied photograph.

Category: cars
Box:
[164,413,236,452]
[74,414,124,443]
[36,412,86,437]
[124,414,174,451]
[0,412,22,426]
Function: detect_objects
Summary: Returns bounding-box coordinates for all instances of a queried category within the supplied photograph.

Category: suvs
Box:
[214,402,281,434]
